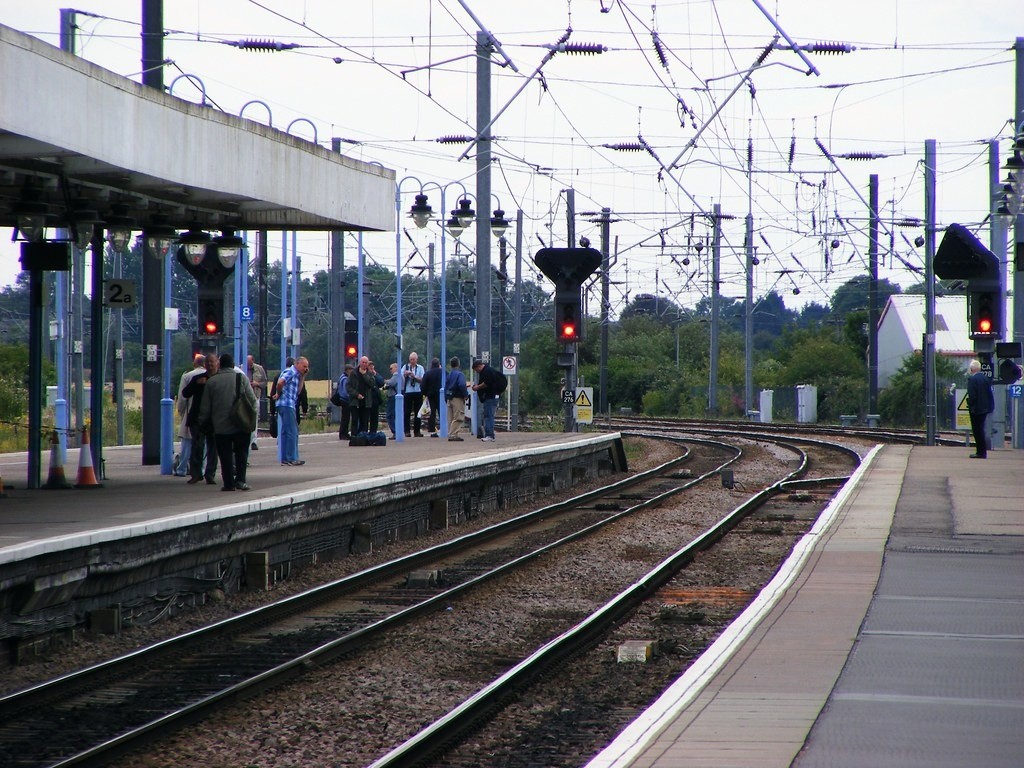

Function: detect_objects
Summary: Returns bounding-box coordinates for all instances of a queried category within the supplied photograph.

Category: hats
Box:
[343,363,355,370]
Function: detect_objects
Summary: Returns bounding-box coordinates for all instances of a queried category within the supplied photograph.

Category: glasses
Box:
[389,367,393,370]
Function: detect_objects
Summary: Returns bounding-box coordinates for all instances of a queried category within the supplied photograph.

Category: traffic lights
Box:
[969,278,1007,339]
[345,332,357,357]
[197,280,224,335]
[554,296,581,343]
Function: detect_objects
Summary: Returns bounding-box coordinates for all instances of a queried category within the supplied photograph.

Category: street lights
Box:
[393,175,510,445]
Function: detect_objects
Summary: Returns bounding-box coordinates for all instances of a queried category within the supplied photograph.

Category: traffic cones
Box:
[42,431,73,489]
[72,430,104,487]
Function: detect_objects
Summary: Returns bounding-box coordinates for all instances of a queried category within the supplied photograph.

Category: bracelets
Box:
[478,386,481,389]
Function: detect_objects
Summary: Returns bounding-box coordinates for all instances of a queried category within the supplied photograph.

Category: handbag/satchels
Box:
[418,398,431,420]
[348,430,387,446]
[489,371,508,395]
[228,393,257,434]
[269,414,278,439]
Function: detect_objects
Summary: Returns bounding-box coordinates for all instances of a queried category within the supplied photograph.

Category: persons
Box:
[174,355,207,476]
[472,361,500,442]
[444,358,469,441]
[401,353,424,437]
[420,358,442,438]
[966,360,995,458]
[200,354,258,492]
[335,364,354,441]
[345,356,385,437]
[240,355,267,450]
[182,354,219,484]
[382,363,404,440]
[270,357,309,467]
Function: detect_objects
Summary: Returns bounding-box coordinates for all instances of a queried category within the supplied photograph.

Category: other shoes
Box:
[389,433,395,440]
[448,435,465,441]
[340,432,350,440]
[404,431,411,438]
[430,432,438,437]
[413,430,423,437]
[251,443,259,449]
[281,460,307,467]
[969,453,987,459]
[188,475,204,484]
[330,392,341,409]
[235,480,252,489]
[205,475,217,484]
[481,435,496,443]
[221,485,236,491]
